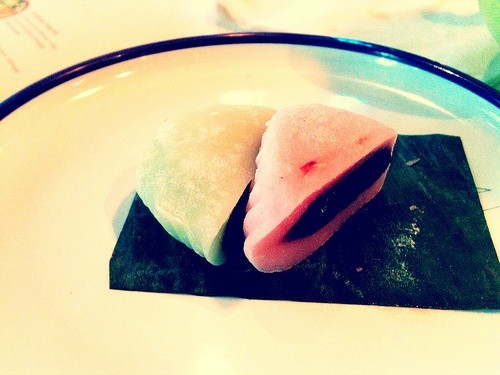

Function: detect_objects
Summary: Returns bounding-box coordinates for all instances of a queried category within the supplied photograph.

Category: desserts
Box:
[132,102,398,274]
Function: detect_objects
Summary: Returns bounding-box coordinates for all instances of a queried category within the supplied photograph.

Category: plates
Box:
[0,31,500,375]
